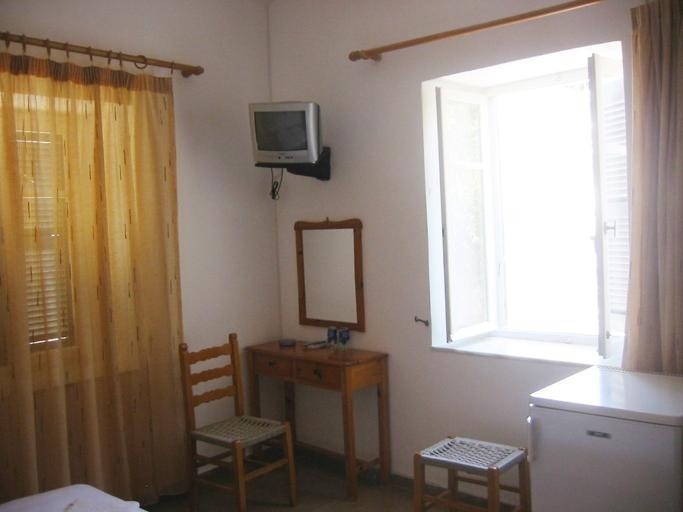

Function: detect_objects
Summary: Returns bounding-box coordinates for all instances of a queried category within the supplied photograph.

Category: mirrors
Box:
[294,217,364,332]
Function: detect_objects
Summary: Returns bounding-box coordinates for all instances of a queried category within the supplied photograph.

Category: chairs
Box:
[179,333,298,512]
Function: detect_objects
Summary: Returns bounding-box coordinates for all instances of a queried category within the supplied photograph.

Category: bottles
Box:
[327,326,338,357]
[338,326,350,359]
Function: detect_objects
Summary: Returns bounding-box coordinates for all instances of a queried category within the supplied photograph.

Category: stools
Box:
[412,436,531,511]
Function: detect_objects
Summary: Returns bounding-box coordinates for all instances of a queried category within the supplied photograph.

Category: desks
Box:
[1,484,148,512]
[244,339,390,501]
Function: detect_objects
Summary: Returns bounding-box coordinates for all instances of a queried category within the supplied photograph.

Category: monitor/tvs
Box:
[249,102,320,162]
